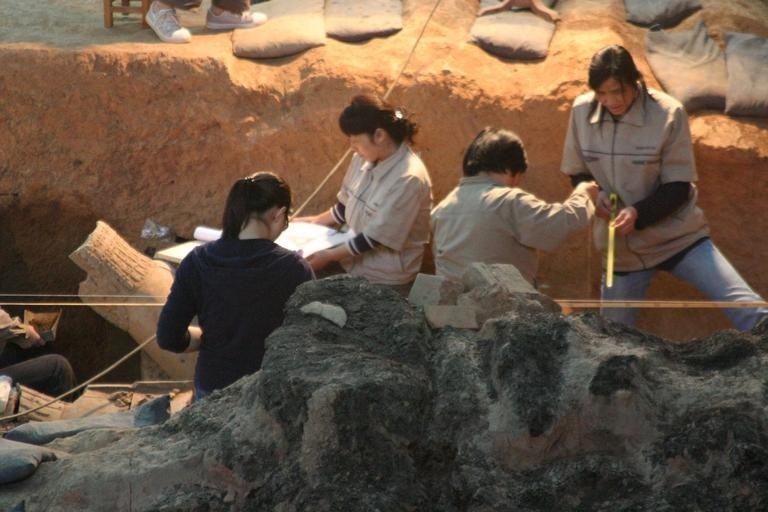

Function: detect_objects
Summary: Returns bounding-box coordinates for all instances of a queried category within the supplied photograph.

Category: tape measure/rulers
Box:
[606,193,618,288]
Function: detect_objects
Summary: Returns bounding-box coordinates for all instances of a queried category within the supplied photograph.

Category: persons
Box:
[0,307,82,403]
[285,94,432,299]
[559,44,768,334]
[145,0,267,43]
[156,171,318,406]
[429,126,599,291]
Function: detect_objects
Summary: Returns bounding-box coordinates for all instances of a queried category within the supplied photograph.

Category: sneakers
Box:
[146,3,189,43]
[204,8,266,30]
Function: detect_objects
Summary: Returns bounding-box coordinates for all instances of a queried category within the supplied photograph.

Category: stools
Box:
[103,0,150,29]
